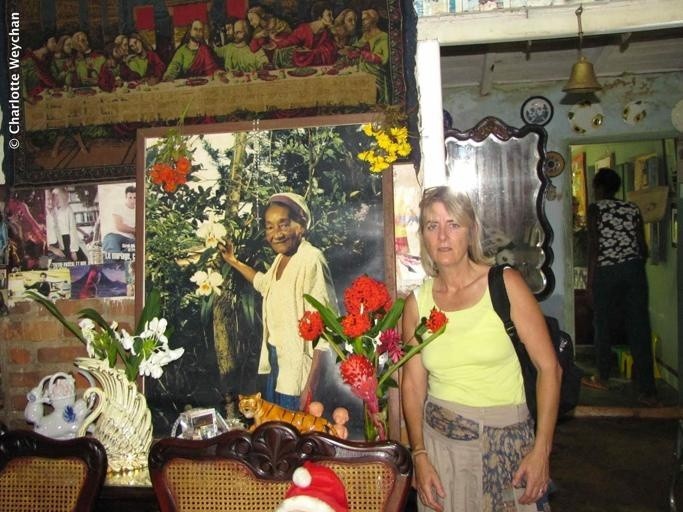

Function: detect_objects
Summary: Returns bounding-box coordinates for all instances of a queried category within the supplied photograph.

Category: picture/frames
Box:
[135,113,400,443]
[594,156,615,173]
[571,152,589,215]
[671,203,679,248]
[0,0,421,194]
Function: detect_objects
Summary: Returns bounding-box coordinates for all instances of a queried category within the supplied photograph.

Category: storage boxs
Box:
[611,344,631,370]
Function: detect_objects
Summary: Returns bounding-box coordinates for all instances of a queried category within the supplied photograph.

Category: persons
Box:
[21,34,56,96]
[103,35,129,67]
[104,186,136,262]
[247,6,294,53]
[224,16,238,40]
[51,35,74,87]
[399,185,563,512]
[45,189,65,253]
[5,187,46,248]
[71,31,116,92]
[329,406,350,440]
[270,2,338,65]
[119,32,164,83]
[351,9,390,70]
[308,402,324,417]
[162,19,225,83]
[220,191,338,414]
[586,166,660,405]
[24,272,51,301]
[210,18,270,73]
[51,187,88,263]
[331,8,359,42]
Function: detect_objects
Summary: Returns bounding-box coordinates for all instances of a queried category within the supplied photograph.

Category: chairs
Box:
[147,422,413,512]
[0,422,109,512]
[618,334,663,378]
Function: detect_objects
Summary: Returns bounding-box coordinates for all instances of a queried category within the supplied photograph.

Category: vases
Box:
[75,357,154,487]
[362,398,389,441]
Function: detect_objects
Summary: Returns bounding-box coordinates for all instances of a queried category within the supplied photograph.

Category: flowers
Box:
[298,272,449,441]
[24,284,185,381]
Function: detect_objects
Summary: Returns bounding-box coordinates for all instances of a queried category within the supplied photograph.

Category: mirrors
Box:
[562,131,683,413]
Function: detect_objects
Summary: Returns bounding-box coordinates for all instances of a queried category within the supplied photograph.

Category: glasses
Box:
[421,187,474,231]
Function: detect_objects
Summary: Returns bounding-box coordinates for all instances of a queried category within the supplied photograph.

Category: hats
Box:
[270,193,311,230]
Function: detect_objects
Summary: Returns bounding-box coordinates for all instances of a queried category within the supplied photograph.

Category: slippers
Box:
[637,392,659,406]
[581,376,609,391]
